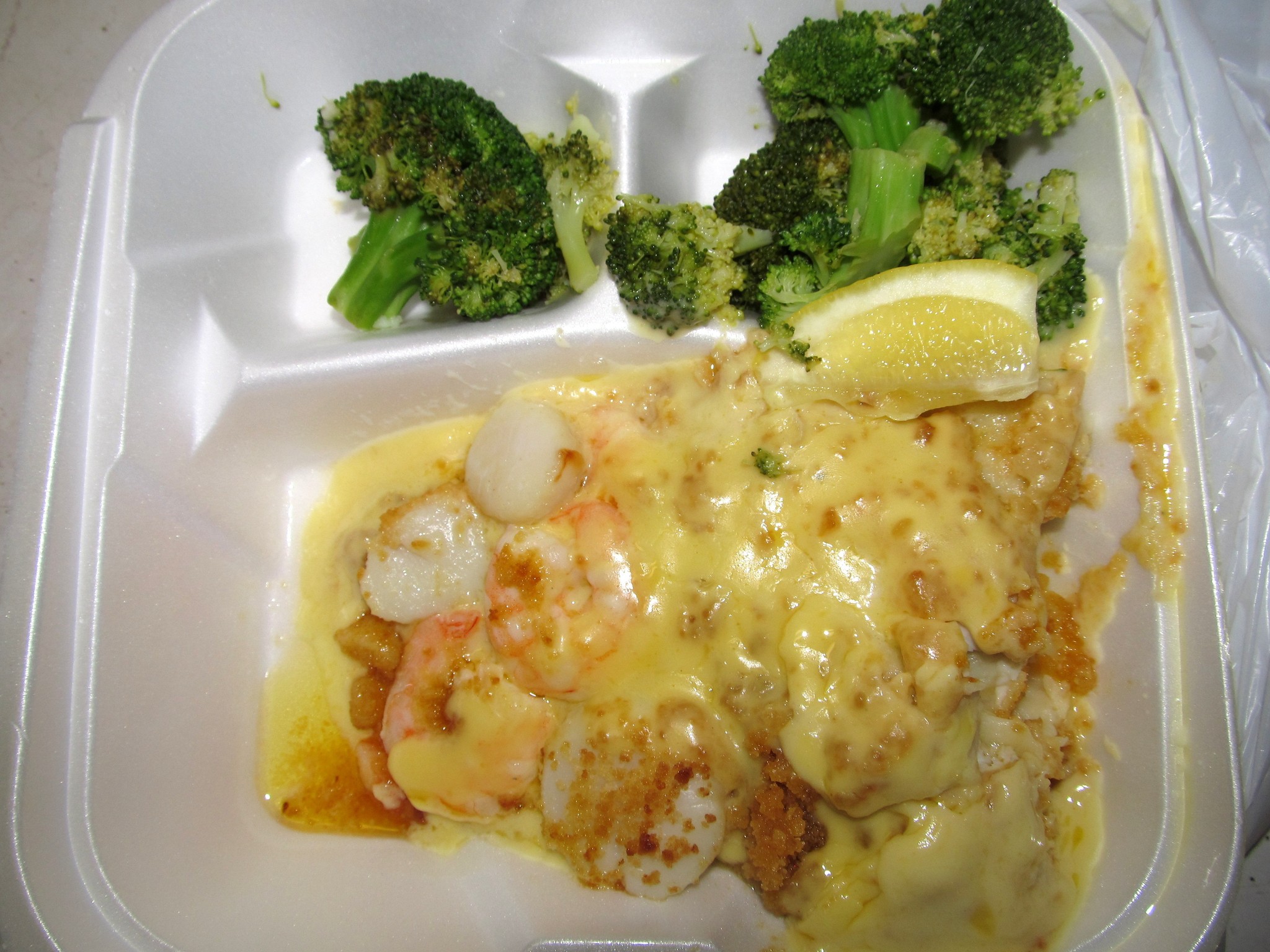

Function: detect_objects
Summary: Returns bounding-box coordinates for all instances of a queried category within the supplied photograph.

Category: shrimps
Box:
[382,497,727,901]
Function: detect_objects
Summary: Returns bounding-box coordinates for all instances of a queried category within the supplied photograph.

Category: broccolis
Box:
[315,0,1090,339]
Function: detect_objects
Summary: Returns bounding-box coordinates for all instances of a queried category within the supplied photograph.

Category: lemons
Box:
[781,259,1041,422]
